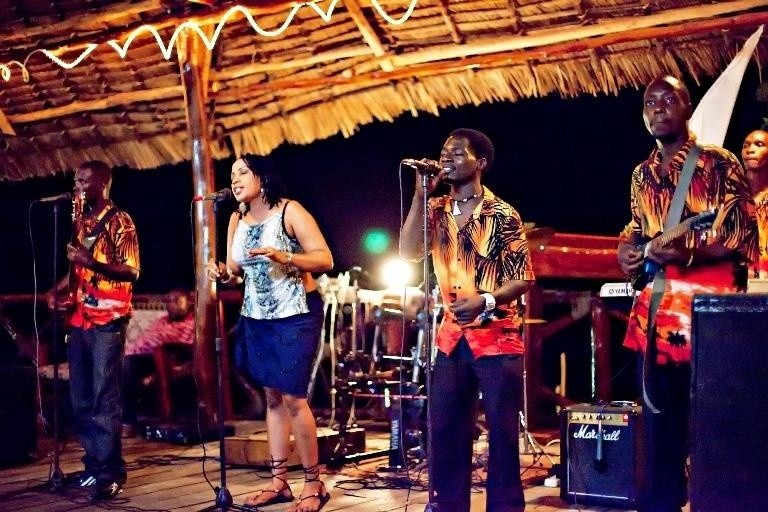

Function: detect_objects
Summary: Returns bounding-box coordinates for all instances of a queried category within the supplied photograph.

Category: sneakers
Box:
[89,482,122,502]
[68,471,95,487]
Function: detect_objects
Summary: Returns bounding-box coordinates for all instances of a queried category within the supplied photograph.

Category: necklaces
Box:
[448,189,483,217]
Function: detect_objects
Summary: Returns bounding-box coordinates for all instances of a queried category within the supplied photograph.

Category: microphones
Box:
[402,155,446,180]
[36,191,71,208]
[192,188,232,203]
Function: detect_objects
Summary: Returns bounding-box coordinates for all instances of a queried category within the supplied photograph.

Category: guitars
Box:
[52,192,87,312]
[626,196,718,266]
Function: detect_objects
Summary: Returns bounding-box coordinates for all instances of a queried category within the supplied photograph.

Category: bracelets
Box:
[220,266,233,284]
[282,252,292,267]
[686,248,694,268]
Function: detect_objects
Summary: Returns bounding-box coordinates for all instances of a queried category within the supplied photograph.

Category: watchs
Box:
[479,292,496,313]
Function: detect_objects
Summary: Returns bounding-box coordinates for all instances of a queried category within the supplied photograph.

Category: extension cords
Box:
[543,473,559,488]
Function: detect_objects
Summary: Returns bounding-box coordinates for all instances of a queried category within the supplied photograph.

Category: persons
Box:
[120,288,196,439]
[617,73,758,512]
[397,128,537,512]
[45,160,142,502]
[741,129,768,294]
[204,152,336,512]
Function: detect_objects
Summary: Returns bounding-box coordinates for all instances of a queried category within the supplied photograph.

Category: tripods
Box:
[0,205,99,502]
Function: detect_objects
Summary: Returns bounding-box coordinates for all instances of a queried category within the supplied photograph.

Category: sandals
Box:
[293,463,331,509]
[244,459,295,507]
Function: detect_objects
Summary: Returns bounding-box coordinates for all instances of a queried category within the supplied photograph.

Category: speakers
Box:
[559,405,644,508]
[690,296,767,512]
